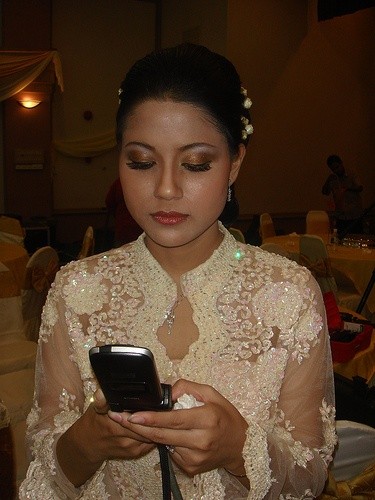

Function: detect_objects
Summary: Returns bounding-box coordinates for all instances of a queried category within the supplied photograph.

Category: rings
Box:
[166,446,175,454]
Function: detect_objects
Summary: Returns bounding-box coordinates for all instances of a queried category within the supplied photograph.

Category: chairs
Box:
[229,209,375,318]
[0,216,94,500]
[320,420,375,500]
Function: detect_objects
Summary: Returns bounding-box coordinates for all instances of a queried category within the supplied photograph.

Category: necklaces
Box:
[167,293,184,333]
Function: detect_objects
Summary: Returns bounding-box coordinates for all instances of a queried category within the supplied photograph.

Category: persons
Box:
[320,154,365,233]
[17,44,340,500]
[105,178,140,245]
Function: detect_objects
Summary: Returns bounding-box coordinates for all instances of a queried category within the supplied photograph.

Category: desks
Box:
[0,241,29,299]
[262,235,375,313]
[325,305,375,388]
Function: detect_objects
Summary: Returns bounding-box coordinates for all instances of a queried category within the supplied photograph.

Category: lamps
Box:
[16,99,43,109]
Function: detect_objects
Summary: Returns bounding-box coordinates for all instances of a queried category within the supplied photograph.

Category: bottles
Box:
[332,229,339,246]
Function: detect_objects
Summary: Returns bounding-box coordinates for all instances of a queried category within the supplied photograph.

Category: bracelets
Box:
[227,470,246,478]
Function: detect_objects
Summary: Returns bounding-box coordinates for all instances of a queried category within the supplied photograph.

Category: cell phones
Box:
[89,345,173,414]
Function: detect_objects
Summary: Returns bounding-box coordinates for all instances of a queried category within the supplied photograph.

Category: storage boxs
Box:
[21,227,51,253]
[321,291,372,364]
